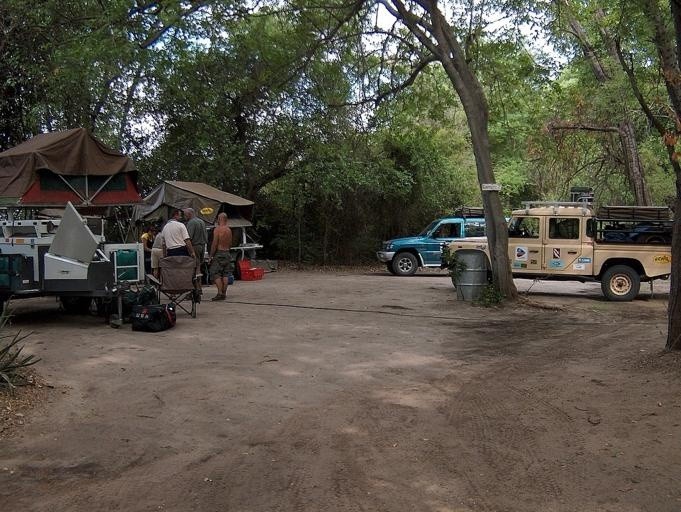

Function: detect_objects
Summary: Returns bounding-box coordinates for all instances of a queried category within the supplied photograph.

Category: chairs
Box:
[145,256,203,317]
[449,228,457,236]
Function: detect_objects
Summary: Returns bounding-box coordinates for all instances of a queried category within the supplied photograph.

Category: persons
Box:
[151,232,165,280]
[140,225,157,285]
[183,207,209,295]
[162,207,197,258]
[207,212,234,301]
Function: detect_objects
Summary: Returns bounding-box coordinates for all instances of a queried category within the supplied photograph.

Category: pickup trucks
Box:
[441,198,673,304]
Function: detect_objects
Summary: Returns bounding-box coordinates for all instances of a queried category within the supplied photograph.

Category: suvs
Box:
[372,212,507,277]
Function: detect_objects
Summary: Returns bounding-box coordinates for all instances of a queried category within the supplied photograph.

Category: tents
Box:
[122,180,257,244]
[0,127,148,207]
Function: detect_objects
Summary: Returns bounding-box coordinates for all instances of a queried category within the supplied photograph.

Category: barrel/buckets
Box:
[448,241,489,301]
